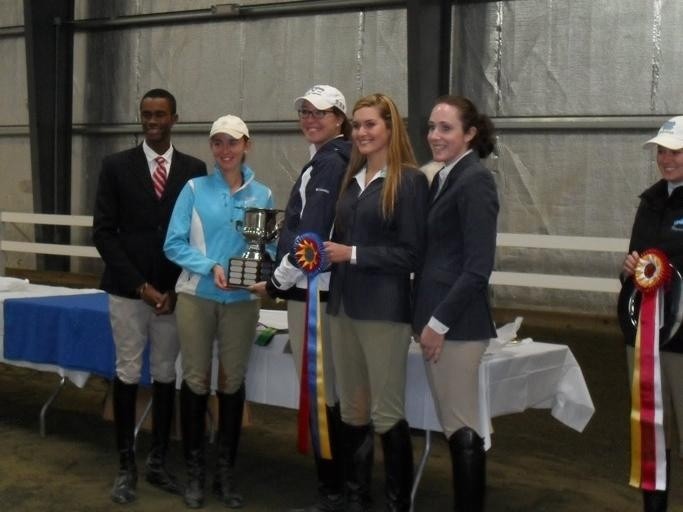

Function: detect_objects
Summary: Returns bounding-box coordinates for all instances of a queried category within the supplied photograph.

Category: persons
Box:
[320,93,429,512]
[247,84,353,509]
[164,114,273,509]
[409,94,500,512]
[91,89,209,506]
[618,113,683,512]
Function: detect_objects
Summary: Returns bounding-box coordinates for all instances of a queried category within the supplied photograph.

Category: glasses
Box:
[298,109,334,119]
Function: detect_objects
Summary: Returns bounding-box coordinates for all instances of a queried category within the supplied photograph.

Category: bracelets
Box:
[136,281,149,299]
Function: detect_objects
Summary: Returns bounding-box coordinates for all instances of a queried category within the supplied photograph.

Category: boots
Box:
[447,426,486,512]
[144,373,245,508]
[310,398,414,512]
[111,376,138,502]
[642,448,670,512]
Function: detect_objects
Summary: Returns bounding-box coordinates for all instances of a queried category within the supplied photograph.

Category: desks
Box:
[0,274,571,510]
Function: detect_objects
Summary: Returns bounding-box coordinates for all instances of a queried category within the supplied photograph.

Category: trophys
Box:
[223,204,287,292]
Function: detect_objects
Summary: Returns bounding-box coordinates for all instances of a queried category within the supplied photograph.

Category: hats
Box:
[641,115,682,151]
[209,114,250,139]
[294,84,347,115]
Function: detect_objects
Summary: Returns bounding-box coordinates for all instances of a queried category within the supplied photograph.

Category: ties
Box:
[152,157,166,199]
